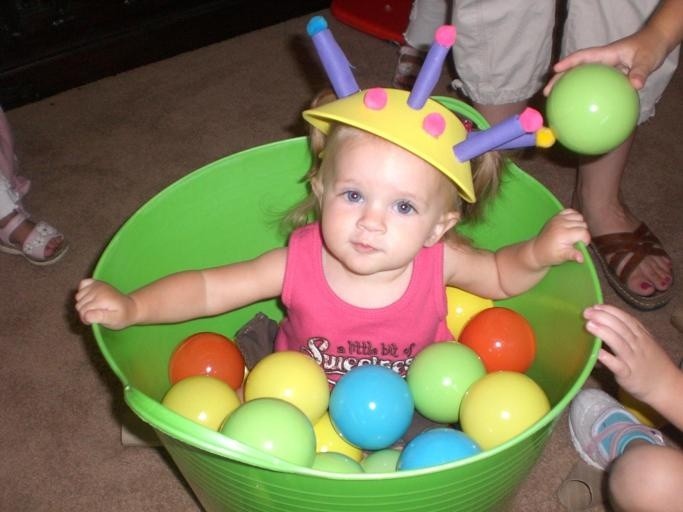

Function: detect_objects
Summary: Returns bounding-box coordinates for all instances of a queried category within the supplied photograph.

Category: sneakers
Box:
[564,382,669,476]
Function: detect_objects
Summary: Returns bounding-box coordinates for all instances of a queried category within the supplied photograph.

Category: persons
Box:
[74,15,591,396]
[0,106,71,266]
[567,303,683,512]
[392,1,453,91]
[451,0,682,312]
[543,0,682,98]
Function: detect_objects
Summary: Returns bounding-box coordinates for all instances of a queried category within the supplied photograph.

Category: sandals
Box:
[1,208,74,268]
[390,45,429,95]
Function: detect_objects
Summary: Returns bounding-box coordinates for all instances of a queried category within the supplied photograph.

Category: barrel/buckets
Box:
[88,95,605,512]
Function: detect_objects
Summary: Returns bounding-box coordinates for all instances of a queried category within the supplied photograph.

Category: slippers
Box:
[571,196,679,313]
